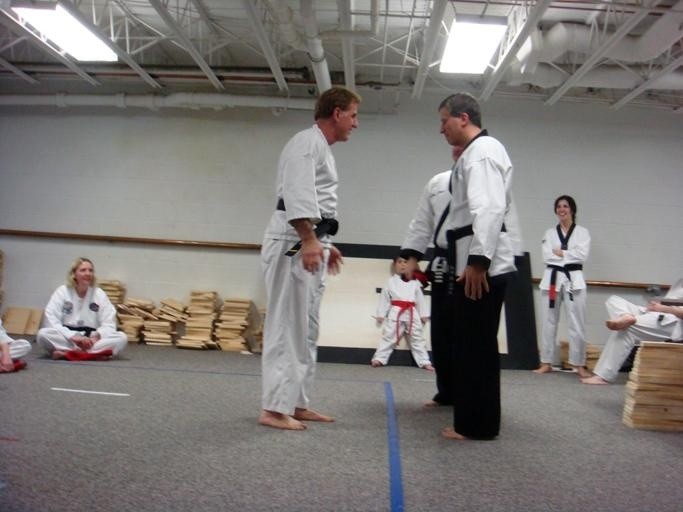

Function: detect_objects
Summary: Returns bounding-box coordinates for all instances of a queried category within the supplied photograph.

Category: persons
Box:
[533,194,592,378]
[0,316,32,374]
[35,256,129,360]
[581,276,683,385]
[436,91,521,440]
[258,88,362,432]
[370,252,436,371]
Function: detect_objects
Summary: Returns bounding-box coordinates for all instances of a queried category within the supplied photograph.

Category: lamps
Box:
[12,1,119,64]
[437,13,509,76]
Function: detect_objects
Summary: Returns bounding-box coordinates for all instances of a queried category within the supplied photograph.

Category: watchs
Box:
[402,144,467,408]
